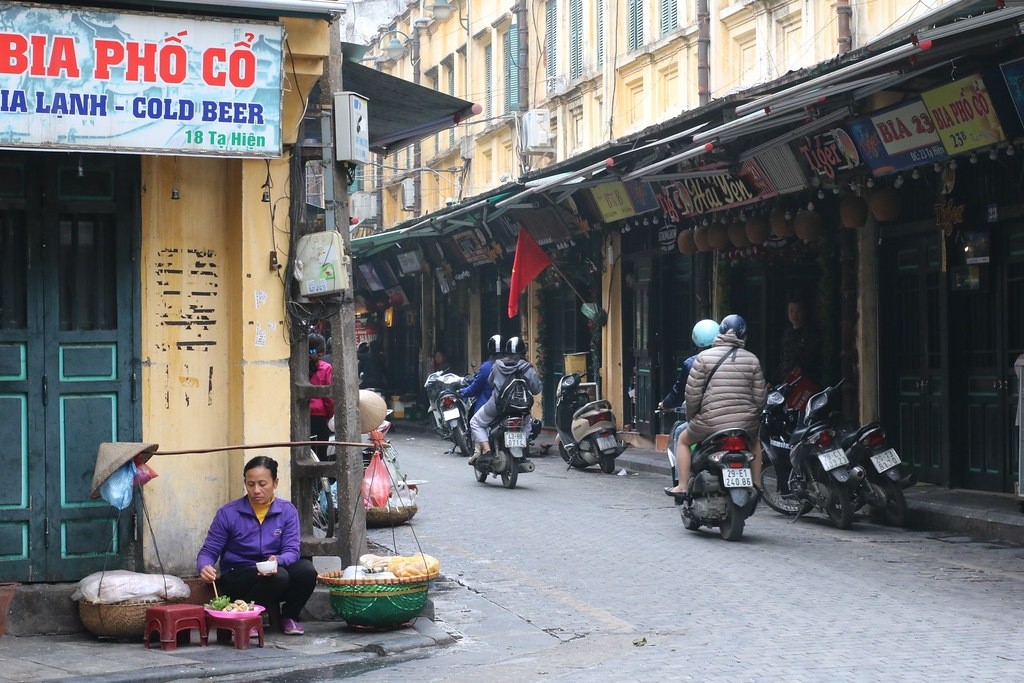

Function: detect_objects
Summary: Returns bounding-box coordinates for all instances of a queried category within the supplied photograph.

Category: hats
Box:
[327,389,387,435]
[90,441,160,501]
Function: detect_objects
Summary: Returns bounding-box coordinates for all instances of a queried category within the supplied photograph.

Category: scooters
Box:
[654,400,766,541]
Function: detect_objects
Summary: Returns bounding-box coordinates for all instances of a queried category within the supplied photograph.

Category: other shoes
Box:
[663,486,688,499]
[468,450,480,465]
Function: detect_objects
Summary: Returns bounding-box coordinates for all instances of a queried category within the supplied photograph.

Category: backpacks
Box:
[494,364,535,417]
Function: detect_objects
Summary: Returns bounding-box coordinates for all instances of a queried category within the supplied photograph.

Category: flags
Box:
[508,226,551,318]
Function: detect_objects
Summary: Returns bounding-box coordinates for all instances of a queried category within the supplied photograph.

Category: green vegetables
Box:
[204,594,231,611]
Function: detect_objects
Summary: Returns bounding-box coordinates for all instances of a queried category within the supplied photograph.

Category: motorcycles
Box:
[554,371,632,474]
[806,378,919,527]
[755,376,876,529]
[455,359,535,489]
[423,359,542,456]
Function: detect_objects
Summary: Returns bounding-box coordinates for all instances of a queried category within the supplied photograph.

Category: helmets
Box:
[506,336,528,356]
[487,335,508,355]
[356,341,369,354]
[691,319,719,348]
[719,314,748,339]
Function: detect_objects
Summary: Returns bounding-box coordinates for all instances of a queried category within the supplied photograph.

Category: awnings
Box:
[306,57,482,157]
[346,0,1024,249]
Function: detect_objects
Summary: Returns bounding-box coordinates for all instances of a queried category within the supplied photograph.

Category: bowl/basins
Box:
[256,561,277,573]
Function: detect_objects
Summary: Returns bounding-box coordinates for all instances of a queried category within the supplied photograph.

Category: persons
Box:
[356,341,392,396]
[309,333,331,460]
[767,299,816,389]
[660,315,769,498]
[460,335,542,464]
[197,455,317,634]
[433,351,452,429]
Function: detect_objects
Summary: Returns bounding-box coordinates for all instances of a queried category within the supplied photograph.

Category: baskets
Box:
[77,595,165,640]
[364,505,418,530]
[316,571,438,632]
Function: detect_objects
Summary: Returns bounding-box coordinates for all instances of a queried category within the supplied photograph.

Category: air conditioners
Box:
[350,193,377,229]
[519,109,551,156]
[401,178,415,209]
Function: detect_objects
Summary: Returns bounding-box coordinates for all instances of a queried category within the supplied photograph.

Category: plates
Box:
[203,604,265,618]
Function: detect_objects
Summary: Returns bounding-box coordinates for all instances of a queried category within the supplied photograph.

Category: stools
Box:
[143,604,265,649]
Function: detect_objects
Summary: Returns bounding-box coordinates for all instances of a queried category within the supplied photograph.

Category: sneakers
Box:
[478,450,493,462]
[249,627,258,637]
[280,617,305,635]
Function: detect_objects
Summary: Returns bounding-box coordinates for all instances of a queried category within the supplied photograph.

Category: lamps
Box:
[423,0,469,37]
[378,28,420,66]
